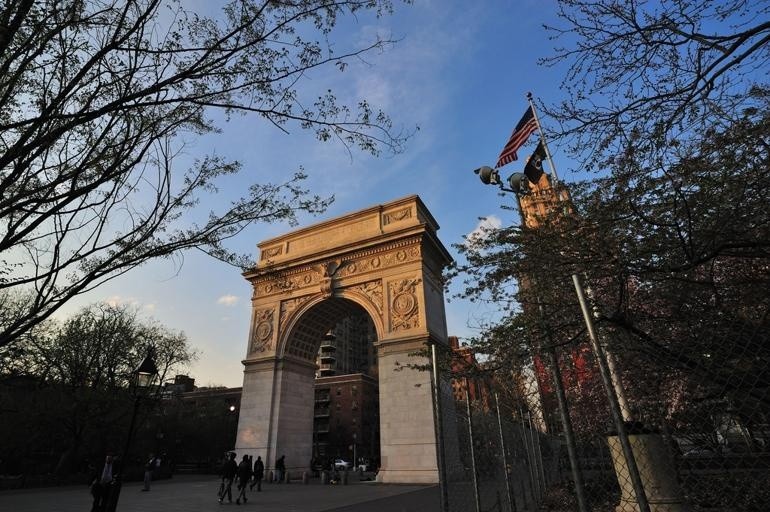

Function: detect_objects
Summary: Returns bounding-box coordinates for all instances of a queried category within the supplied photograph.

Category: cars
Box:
[330,459,352,470]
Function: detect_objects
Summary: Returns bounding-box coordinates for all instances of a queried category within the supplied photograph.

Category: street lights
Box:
[120,350,158,474]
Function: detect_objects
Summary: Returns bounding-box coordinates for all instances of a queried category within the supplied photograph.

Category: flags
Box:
[523,142,547,185]
[492,105,538,171]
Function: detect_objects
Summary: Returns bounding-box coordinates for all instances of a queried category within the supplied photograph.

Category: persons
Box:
[250,455,265,492]
[234,454,248,490]
[217,451,238,505]
[142,453,157,492]
[91,453,124,511]
[235,454,251,505]
[275,454,285,483]
